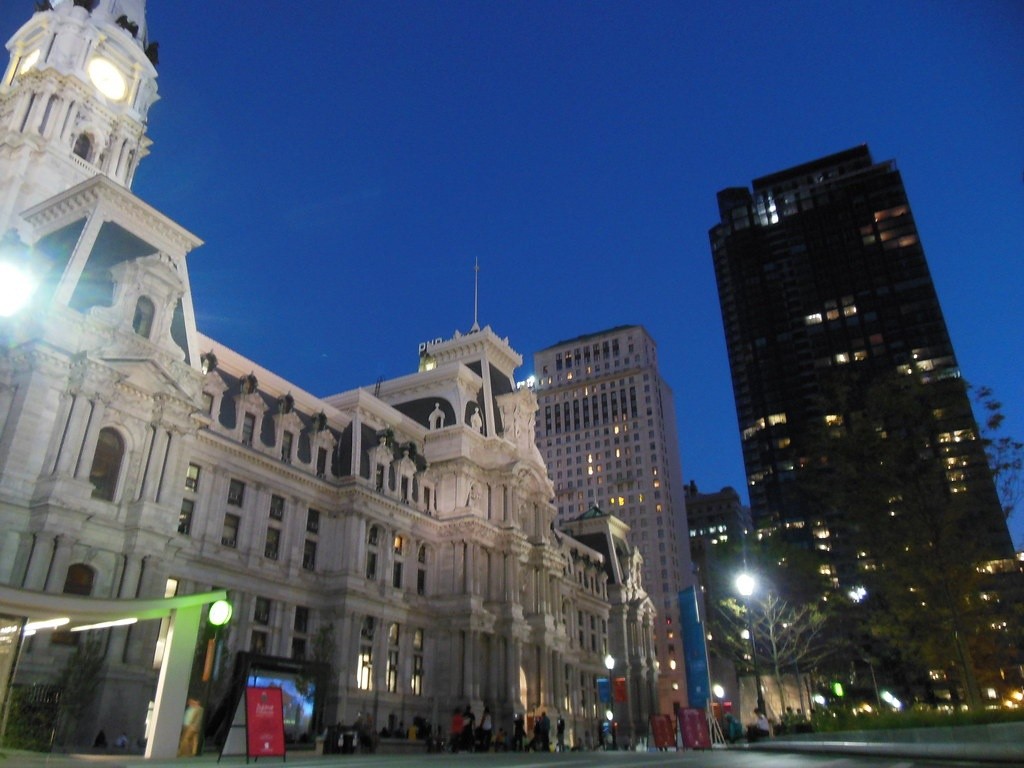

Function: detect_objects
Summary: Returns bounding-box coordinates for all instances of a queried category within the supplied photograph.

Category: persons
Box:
[514,406,522,439]
[747,708,769,744]
[178,694,203,756]
[787,706,816,723]
[116,732,128,748]
[529,413,535,449]
[379,703,633,756]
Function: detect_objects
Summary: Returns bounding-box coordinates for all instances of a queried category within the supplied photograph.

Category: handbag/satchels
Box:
[462,718,471,726]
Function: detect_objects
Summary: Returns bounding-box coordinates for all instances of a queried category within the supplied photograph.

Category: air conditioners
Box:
[371,536,377,544]
[426,510,432,517]
[266,550,273,557]
[282,456,289,464]
[366,628,374,637]
[378,488,385,495]
[306,561,313,569]
[369,574,375,579]
[180,525,190,535]
[402,499,409,506]
[273,509,282,518]
[315,472,323,480]
[242,439,250,446]
[186,478,195,488]
[308,521,319,531]
[223,538,236,548]
[394,547,400,552]
[226,492,239,502]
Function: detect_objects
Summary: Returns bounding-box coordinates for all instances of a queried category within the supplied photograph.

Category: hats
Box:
[723,713,733,719]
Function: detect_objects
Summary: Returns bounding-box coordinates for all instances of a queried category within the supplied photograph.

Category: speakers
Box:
[674,702,680,713]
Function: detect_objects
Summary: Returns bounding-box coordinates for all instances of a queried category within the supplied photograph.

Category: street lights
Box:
[711,683,728,741]
[606,653,618,751]
[736,574,769,719]
[195,601,233,755]
